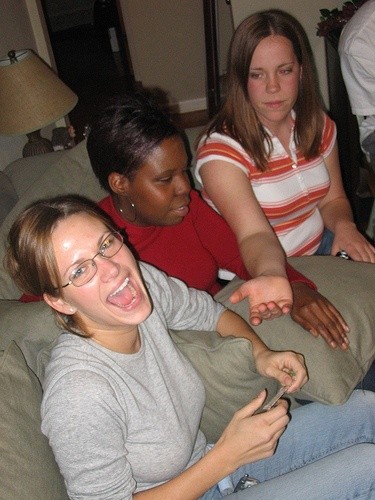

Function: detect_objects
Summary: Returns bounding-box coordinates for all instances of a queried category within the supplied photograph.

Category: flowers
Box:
[314,0,364,47]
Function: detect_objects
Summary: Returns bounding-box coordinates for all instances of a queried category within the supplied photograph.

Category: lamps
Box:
[0,49,78,157]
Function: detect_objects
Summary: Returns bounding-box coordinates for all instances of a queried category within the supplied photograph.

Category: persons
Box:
[338,0,375,173]
[195,8,374,327]
[19,92,375,407]
[2,193,375,500]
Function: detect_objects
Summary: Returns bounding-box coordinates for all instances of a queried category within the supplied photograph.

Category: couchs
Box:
[0,125,206,500]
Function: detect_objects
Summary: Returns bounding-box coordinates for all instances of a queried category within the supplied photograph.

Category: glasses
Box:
[44,232,124,290]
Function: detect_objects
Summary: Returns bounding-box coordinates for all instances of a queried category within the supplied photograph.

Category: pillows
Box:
[168,328,287,446]
[212,256,375,407]
